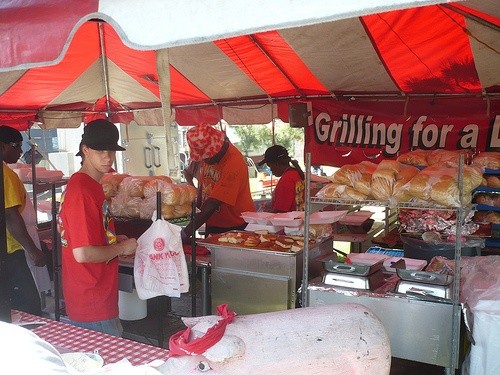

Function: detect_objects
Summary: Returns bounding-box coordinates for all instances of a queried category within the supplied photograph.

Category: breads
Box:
[218,232,315,253]
[315,148,500,225]
[101,174,198,220]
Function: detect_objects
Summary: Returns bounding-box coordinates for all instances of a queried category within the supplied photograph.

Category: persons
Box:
[181,121,256,243]
[0,125,52,317]
[57,118,138,338]
[258,145,306,214]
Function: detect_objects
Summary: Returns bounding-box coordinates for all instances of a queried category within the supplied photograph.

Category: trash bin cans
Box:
[400,230,481,271]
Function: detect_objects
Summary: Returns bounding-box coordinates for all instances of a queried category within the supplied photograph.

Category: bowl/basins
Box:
[347,219,375,234]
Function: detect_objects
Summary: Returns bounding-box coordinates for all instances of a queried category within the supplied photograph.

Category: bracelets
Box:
[181,230,189,240]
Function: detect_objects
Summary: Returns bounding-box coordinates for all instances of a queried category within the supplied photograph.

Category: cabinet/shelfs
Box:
[303,149,500,375]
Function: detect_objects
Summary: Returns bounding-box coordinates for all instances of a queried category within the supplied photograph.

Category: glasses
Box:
[5,141,23,148]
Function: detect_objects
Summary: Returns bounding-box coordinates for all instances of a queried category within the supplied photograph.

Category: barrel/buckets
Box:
[400,233,482,263]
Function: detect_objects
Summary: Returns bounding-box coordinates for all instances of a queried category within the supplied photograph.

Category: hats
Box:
[186,122,225,161]
[257,145,288,167]
[83,120,126,153]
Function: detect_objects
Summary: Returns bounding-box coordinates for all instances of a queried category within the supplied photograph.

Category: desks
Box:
[333,222,384,253]
[57,239,211,350]
[11,309,169,366]
